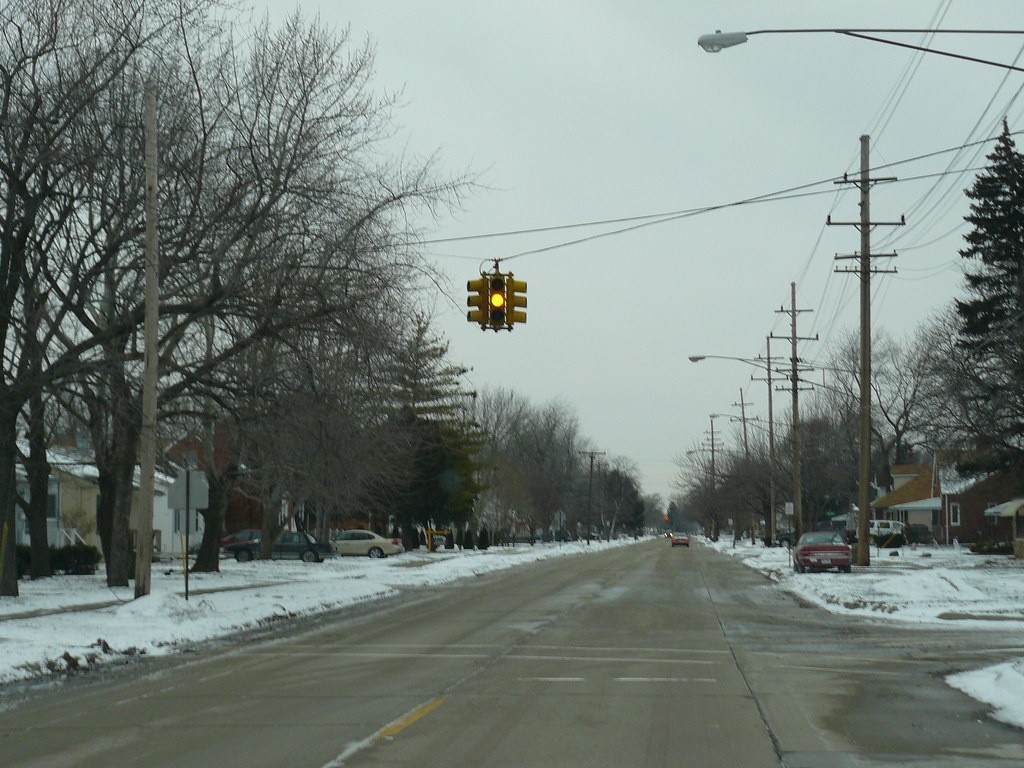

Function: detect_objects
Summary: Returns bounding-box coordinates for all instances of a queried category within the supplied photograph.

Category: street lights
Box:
[709,412,756,545]
[688,354,803,545]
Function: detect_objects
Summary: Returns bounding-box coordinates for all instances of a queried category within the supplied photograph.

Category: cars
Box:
[854,518,909,546]
[217,528,267,561]
[760,526,798,548]
[792,530,852,574]
[232,531,320,563]
[329,529,406,558]
[671,532,690,548]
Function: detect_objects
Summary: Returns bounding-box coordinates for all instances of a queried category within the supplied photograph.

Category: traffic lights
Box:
[467,278,489,325]
[490,276,505,326]
[505,277,527,325]
